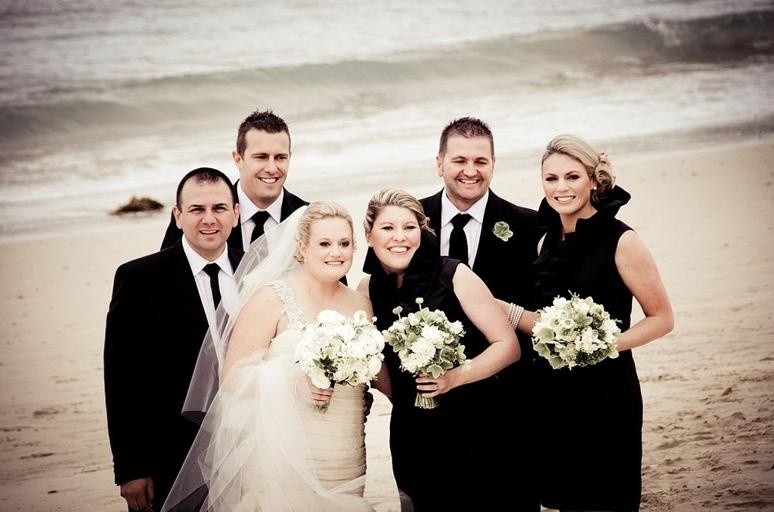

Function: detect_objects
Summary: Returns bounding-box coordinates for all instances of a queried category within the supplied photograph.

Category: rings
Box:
[436,384,439,390]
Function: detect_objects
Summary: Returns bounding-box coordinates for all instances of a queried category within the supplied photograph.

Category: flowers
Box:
[531,289,622,371]
[381,297,472,409]
[297,309,385,414]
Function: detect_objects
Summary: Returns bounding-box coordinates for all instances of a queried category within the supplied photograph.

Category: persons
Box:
[218,200,393,512]
[354,187,525,512]
[491,132,676,511]
[159,108,349,292]
[359,113,539,302]
[101,165,376,512]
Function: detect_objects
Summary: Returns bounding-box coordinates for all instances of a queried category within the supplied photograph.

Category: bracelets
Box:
[293,380,298,398]
[506,301,525,332]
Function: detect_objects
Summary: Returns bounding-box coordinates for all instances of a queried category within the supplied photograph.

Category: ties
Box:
[204,262,232,355]
[448,216,469,273]
[251,213,274,273]
